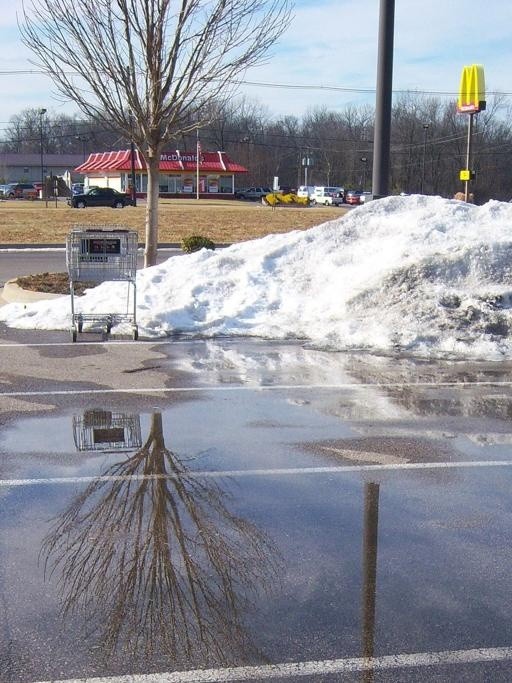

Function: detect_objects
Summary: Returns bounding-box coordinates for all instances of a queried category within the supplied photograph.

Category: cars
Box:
[234,185,373,206]
[72,187,133,210]
[0,181,84,198]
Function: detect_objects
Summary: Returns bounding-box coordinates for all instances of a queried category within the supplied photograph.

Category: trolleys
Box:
[68,408,147,453]
[63,226,140,343]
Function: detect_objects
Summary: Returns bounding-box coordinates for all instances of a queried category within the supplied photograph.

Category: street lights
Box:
[421,121,431,193]
[360,156,367,190]
[39,108,47,190]
[76,135,90,162]
